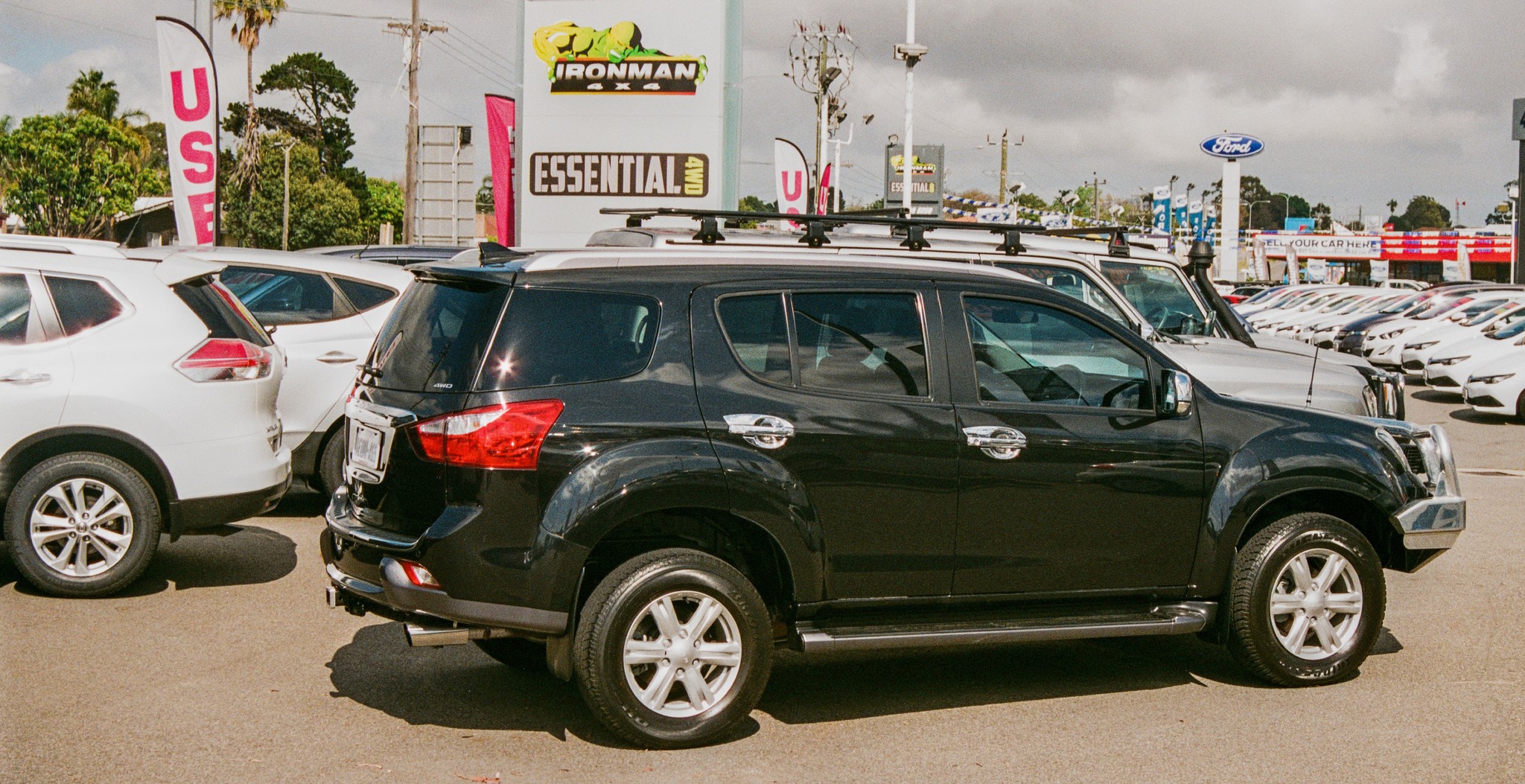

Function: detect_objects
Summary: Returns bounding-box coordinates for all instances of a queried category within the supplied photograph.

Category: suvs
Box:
[586,208,1379,419]
[318,241,1466,751]
[1,234,294,600]
[789,206,1406,418]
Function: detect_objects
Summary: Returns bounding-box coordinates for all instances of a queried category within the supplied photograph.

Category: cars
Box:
[1212,278,1525,423]
[166,246,420,507]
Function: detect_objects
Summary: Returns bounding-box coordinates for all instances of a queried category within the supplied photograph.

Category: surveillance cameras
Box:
[898,44,927,61]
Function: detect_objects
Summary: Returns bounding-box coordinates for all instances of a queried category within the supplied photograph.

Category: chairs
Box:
[871,310,930,398]
[816,306,874,394]
